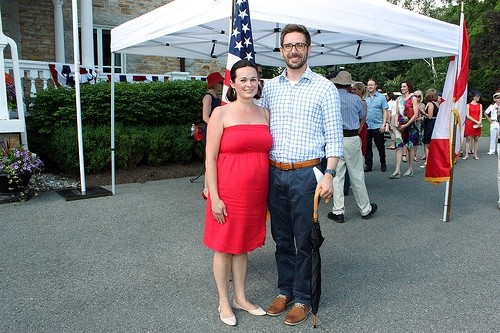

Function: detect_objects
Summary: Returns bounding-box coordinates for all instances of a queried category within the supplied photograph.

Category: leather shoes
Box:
[283,302,312,325]
[266,294,294,316]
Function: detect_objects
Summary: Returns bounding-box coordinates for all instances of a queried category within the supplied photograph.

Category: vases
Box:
[0,174,32,191]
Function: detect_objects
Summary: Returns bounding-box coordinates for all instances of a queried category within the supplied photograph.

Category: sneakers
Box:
[327,212,343,223]
[362,203,377,219]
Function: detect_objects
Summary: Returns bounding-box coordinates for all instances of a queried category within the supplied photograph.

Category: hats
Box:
[493,93,500,98]
[207,72,224,88]
[330,71,357,85]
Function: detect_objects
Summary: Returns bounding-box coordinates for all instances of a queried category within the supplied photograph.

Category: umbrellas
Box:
[310,187,330,327]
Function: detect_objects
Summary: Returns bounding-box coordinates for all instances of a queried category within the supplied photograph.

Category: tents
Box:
[111,0,460,221]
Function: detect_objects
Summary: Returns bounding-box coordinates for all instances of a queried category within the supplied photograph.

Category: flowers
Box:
[0,134,44,198]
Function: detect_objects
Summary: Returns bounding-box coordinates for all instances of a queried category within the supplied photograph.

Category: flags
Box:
[221,0,255,106]
[425,25,470,183]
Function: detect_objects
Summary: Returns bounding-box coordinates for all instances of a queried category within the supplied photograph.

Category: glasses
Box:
[283,43,307,51]
[218,82,223,85]
[414,95,421,98]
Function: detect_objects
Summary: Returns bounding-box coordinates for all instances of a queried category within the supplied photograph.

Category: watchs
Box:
[325,169,336,177]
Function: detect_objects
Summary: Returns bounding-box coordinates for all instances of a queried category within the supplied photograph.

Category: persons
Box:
[351,80,440,178]
[485,93,500,208]
[204,59,273,325]
[253,64,262,106]
[459,91,483,159]
[201,72,225,199]
[328,71,377,223]
[259,24,344,325]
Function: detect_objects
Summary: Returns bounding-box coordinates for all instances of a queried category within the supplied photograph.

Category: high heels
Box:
[390,171,400,178]
[404,170,413,176]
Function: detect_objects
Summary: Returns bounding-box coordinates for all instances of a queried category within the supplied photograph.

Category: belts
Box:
[269,158,320,170]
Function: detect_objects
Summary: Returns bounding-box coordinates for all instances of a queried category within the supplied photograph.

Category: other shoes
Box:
[414,155,417,160]
[402,155,407,161]
[463,156,469,159]
[386,147,395,150]
[421,163,426,167]
[381,162,386,170]
[232,299,266,316]
[487,152,495,155]
[420,156,426,160]
[473,155,479,160]
[218,305,237,326]
[364,166,372,172]
